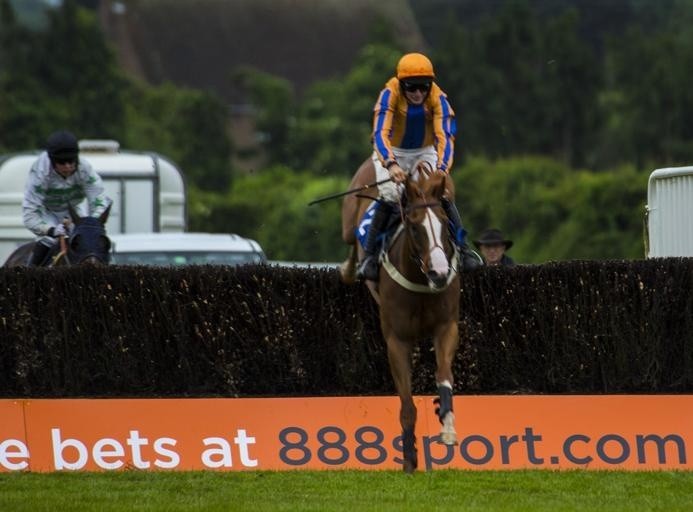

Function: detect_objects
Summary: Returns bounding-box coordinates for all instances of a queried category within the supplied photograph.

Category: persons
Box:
[353,51,483,280]
[20,132,111,265]
[473,227,514,265]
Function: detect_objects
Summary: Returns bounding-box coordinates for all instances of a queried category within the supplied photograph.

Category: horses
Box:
[3,198,117,265]
[336,153,468,475]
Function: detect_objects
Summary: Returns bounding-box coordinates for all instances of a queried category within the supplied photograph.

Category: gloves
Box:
[48,224,65,237]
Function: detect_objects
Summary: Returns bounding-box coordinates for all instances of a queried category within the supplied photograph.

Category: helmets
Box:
[47,131,77,163]
[397,53,434,79]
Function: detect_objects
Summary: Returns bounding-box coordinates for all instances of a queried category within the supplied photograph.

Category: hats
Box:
[473,230,513,250]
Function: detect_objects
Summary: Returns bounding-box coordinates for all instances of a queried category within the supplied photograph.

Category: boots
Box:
[361,200,393,282]
[445,200,480,273]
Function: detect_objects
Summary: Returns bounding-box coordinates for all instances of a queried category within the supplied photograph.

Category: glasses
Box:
[401,80,429,92]
